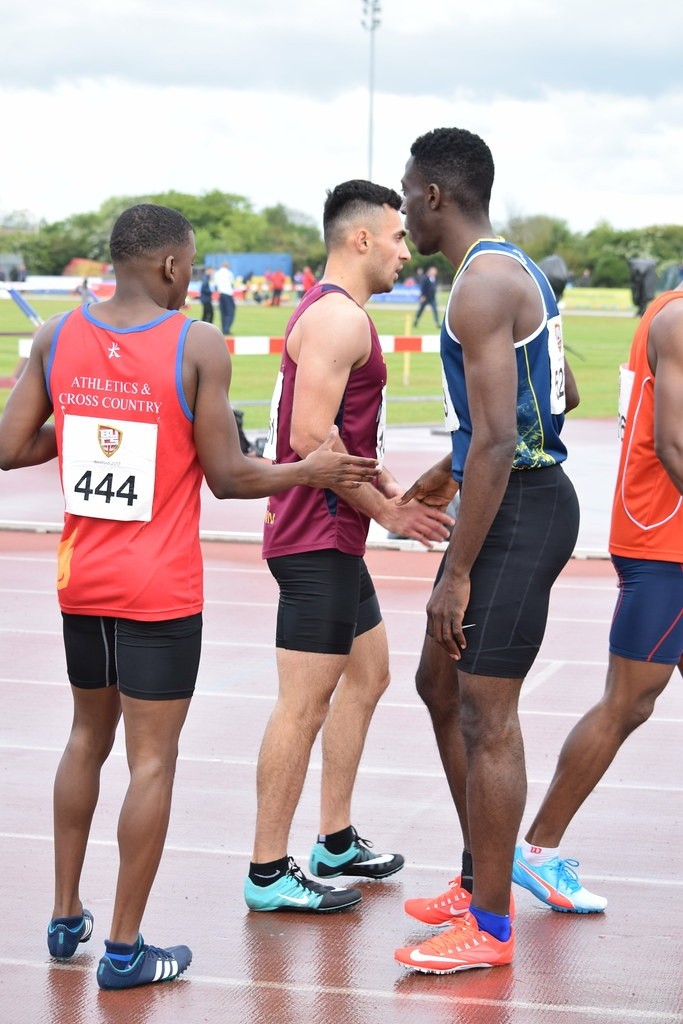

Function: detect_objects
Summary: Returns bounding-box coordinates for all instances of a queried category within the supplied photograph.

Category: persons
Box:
[412,265,444,328]
[201,259,316,336]
[0,205,382,989]
[512,280,683,914]
[393,128,580,976]
[244,179,457,913]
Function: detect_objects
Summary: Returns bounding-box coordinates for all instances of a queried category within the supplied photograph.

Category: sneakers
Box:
[394,911,515,974]
[511,845,607,913]
[404,875,516,926]
[97,933,193,990]
[47,909,94,961]
[309,825,404,879]
[244,856,362,914]
